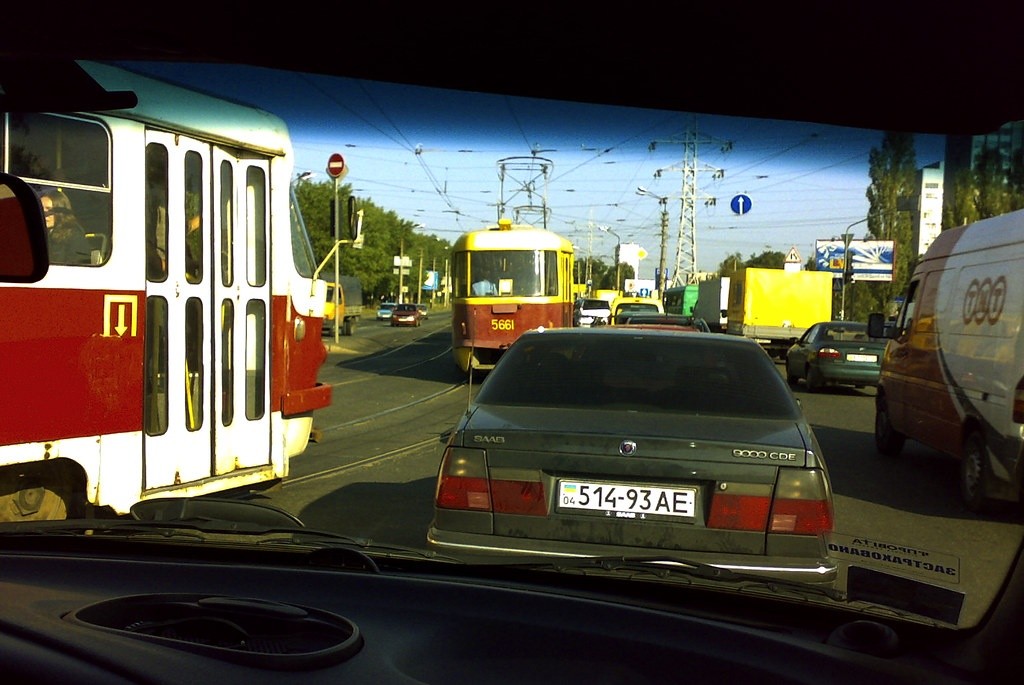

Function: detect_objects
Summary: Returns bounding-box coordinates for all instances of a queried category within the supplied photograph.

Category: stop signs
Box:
[328,153,343,178]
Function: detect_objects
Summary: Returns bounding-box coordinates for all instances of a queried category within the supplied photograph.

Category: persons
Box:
[146,175,202,389]
[37,189,91,265]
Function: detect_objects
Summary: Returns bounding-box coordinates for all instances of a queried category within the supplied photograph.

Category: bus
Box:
[1,59,366,528]
[662,285,700,315]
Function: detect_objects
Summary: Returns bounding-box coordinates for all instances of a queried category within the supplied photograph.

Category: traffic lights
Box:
[896,196,911,211]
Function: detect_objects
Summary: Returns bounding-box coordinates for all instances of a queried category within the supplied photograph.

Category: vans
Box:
[610,297,664,324]
[866,207,1024,508]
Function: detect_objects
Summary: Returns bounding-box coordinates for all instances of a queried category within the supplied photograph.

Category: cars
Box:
[414,304,429,320]
[390,303,421,327]
[589,311,712,335]
[428,327,839,592]
[572,298,612,328]
[785,320,886,393]
[376,303,398,320]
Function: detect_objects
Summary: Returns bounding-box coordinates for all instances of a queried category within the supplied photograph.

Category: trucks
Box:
[689,277,731,334]
[719,268,834,360]
[321,272,362,336]
[590,289,624,308]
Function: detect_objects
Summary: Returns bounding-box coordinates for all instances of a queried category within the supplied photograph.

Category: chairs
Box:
[677,367,732,393]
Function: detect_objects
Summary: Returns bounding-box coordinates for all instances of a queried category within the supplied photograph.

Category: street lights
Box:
[634,185,669,302]
[572,245,592,294]
[429,246,448,309]
[598,226,621,290]
[398,223,427,306]
[417,238,440,305]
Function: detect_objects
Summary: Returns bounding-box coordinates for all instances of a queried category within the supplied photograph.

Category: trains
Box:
[456,218,574,371]
